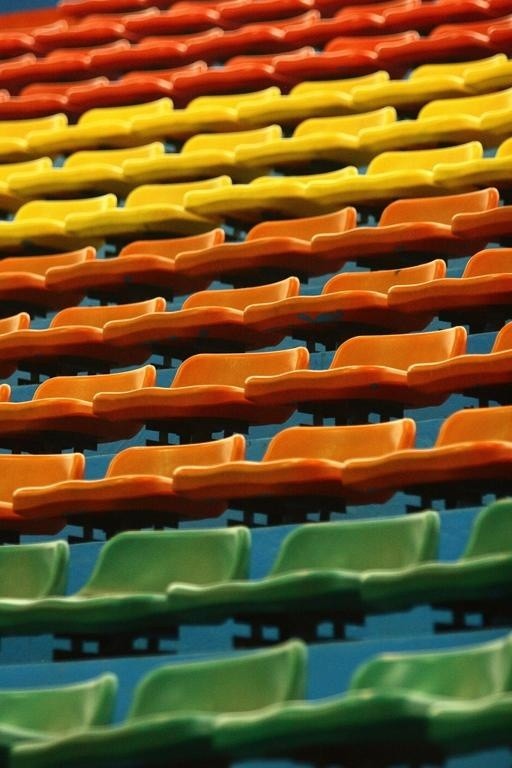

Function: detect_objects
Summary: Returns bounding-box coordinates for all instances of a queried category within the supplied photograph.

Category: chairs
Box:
[0,0,512,768]
